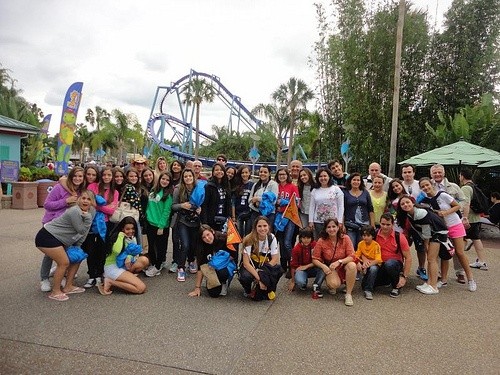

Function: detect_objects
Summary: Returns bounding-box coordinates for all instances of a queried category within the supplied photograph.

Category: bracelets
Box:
[401,273,407,278]
[338,259,342,265]
[195,285,200,289]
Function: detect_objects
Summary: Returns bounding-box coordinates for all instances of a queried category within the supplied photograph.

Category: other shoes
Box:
[455,275,466,284]
[390,288,400,298]
[364,290,373,299]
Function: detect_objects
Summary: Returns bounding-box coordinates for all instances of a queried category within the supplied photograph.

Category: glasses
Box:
[218,158,225,162]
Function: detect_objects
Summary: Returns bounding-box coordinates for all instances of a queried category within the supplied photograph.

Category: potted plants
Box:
[11,166,38,210]
[30,166,59,206]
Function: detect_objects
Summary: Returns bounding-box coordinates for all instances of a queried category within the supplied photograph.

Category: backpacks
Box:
[468,181,488,214]
[438,236,455,260]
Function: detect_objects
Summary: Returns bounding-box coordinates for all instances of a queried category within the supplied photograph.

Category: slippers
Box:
[468,280,476,291]
[345,293,354,306]
[64,286,85,294]
[329,289,336,294]
[416,283,439,294]
[48,292,69,302]
[437,281,447,287]
[97,282,112,296]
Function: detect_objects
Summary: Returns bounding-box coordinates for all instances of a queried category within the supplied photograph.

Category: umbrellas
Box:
[397,135,500,186]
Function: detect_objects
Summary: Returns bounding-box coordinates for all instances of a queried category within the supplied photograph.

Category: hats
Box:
[132,156,148,167]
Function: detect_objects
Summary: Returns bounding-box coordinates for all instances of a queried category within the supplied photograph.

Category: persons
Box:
[34,156,500,306]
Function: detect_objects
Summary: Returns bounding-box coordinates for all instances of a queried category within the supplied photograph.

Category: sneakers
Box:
[169,263,178,273]
[177,268,186,282]
[143,264,162,277]
[40,278,51,291]
[84,279,101,288]
[312,283,324,298]
[470,258,487,270]
[188,262,197,273]
[61,277,66,288]
[464,239,473,251]
[416,266,428,280]
